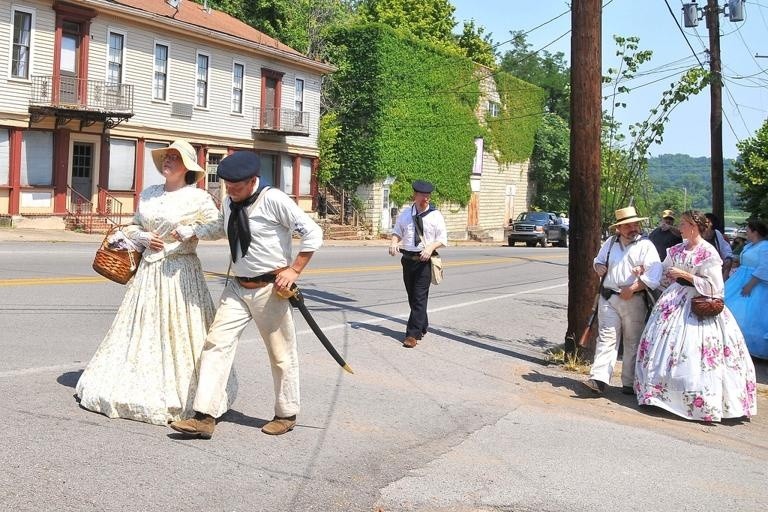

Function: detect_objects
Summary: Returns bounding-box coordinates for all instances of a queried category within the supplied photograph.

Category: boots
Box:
[581,379,606,395]
[622,385,634,393]
[262,414,297,434]
[169,411,215,439]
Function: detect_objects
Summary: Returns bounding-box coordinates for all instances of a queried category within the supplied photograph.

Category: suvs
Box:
[507,212,568,247]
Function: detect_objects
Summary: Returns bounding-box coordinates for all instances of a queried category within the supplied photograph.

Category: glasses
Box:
[158,152,182,160]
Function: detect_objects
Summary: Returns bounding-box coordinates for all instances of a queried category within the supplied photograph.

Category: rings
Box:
[277,277,280,280]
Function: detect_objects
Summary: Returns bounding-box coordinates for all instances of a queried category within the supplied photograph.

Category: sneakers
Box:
[403,336,417,347]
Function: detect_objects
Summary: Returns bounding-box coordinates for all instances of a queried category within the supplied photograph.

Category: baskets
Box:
[92,222,147,284]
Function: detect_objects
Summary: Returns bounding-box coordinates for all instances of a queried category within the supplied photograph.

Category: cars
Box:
[724,228,738,239]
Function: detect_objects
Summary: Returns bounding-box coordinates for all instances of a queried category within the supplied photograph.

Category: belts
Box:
[402,249,424,256]
[230,272,276,285]
[603,289,642,296]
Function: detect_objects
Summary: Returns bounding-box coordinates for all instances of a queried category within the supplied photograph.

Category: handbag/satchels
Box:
[643,289,662,310]
[691,280,724,316]
[429,253,445,284]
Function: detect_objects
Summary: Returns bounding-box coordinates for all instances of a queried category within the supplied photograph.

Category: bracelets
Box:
[290,266,298,274]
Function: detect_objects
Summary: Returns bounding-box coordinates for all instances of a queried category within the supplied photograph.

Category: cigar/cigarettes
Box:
[425,200,430,204]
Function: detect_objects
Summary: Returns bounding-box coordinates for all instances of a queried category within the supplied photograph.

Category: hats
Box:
[662,209,674,219]
[218,151,261,182]
[607,207,648,228]
[150,138,206,186]
[413,180,433,192]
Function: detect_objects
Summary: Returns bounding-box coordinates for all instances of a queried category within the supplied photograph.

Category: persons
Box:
[387,178,448,349]
[700,212,734,281]
[647,208,682,263]
[629,209,758,424]
[580,205,662,397]
[72,137,240,428]
[717,219,768,363]
[167,150,324,441]
